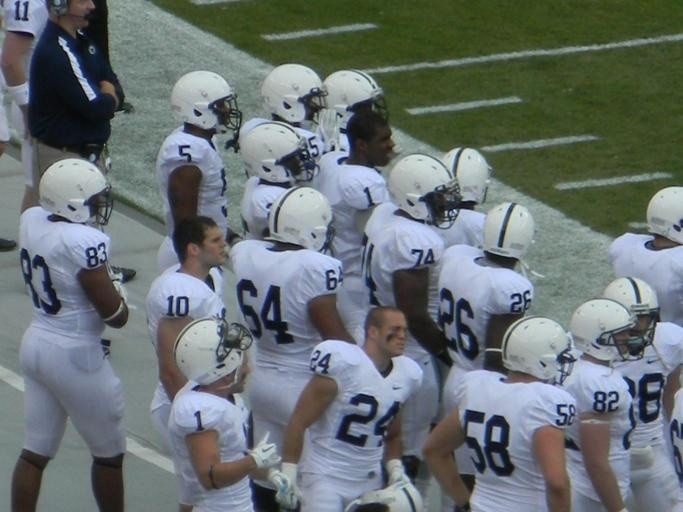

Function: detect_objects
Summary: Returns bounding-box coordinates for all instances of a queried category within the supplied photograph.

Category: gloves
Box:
[629,445,655,471]
[112,278,132,314]
[243,430,283,474]
[261,466,306,511]
[383,458,410,488]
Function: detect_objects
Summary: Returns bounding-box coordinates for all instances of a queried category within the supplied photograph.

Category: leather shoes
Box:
[0,235,17,253]
[108,262,137,283]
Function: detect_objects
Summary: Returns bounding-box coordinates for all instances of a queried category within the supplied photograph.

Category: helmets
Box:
[442,146,494,206]
[239,121,309,184]
[343,479,425,512]
[569,275,661,362]
[37,158,111,223]
[260,62,328,125]
[481,202,537,264]
[168,70,238,131]
[316,67,385,117]
[644,185,682,249]
[384,153,459,221]
[500,314,572,381]
[172,315,242,388]
[263,183,335,252]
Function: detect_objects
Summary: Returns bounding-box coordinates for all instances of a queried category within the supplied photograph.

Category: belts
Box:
[33,132,105,162]
